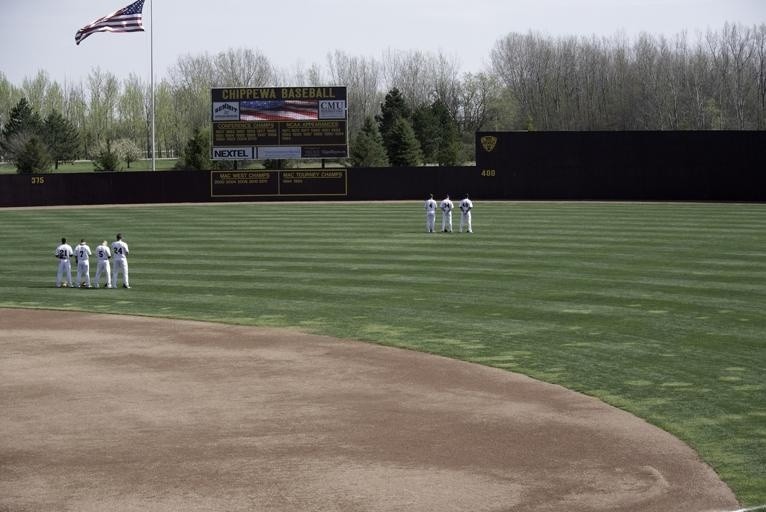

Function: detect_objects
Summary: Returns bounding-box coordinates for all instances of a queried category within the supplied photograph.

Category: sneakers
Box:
[57,283,130,288]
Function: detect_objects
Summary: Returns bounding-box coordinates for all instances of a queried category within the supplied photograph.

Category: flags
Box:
[239,100,318,120]
[75,0,145,45]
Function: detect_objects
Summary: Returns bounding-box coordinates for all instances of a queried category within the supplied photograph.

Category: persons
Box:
[112,233,132,288]
[425,194,437,233]
[440,195,454,233]
[55,238,74,287]
[93,240,112,288]
[74,239,92,288]
[459,193,474,232]
